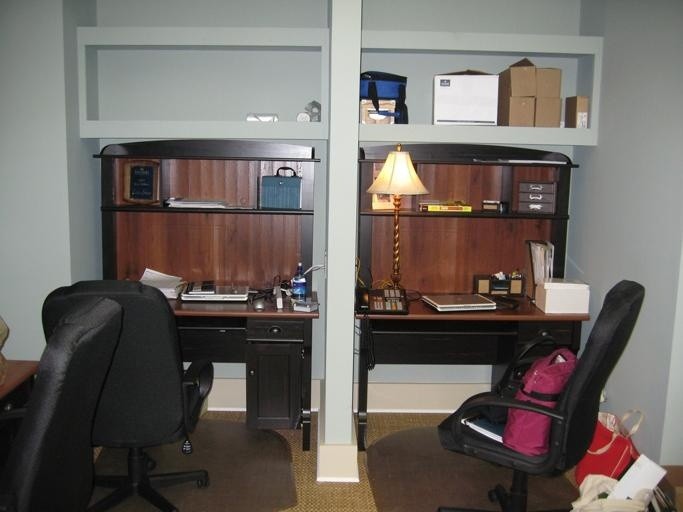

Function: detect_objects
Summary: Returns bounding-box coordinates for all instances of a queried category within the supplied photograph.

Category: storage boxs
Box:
[432,55,593,136]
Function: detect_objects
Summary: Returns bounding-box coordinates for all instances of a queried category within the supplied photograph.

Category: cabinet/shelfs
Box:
[361,142,581,309]
[100,144,316,289]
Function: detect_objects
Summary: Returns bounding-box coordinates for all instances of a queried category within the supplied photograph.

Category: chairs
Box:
[64,282,211,511]
[437,279,645,509]
[2,298,126,510]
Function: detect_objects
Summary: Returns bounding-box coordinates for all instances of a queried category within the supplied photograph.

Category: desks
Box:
[172,291,306,441]
[361,292,577,451]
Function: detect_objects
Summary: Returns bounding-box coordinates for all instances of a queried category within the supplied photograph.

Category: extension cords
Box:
[274,285,284,309]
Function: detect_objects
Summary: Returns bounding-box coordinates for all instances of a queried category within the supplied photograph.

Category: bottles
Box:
[290,261,307,304]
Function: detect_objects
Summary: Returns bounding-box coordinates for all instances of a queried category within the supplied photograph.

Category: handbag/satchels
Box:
[360,71,408,123]
[501,347,576,459]
[568,473,655,512]
[563,409,645,490]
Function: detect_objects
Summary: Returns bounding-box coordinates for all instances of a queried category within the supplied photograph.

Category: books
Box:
[459,414,506,444]
[530,240,554,285]
[187,280,215,294]
[165,196,228,208]
[419,200,472,213]
[138,267,183,300]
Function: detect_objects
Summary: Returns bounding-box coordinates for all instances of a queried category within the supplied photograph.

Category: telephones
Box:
[357,288,408,314]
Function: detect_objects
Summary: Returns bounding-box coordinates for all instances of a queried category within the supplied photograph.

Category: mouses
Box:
[252,299,264,312]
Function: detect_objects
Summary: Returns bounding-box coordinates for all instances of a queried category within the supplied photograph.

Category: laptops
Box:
[422,293,497,312]
[181,285,249,302]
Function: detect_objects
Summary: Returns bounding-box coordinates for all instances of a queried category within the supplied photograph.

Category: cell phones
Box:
[492,294,521,310]
